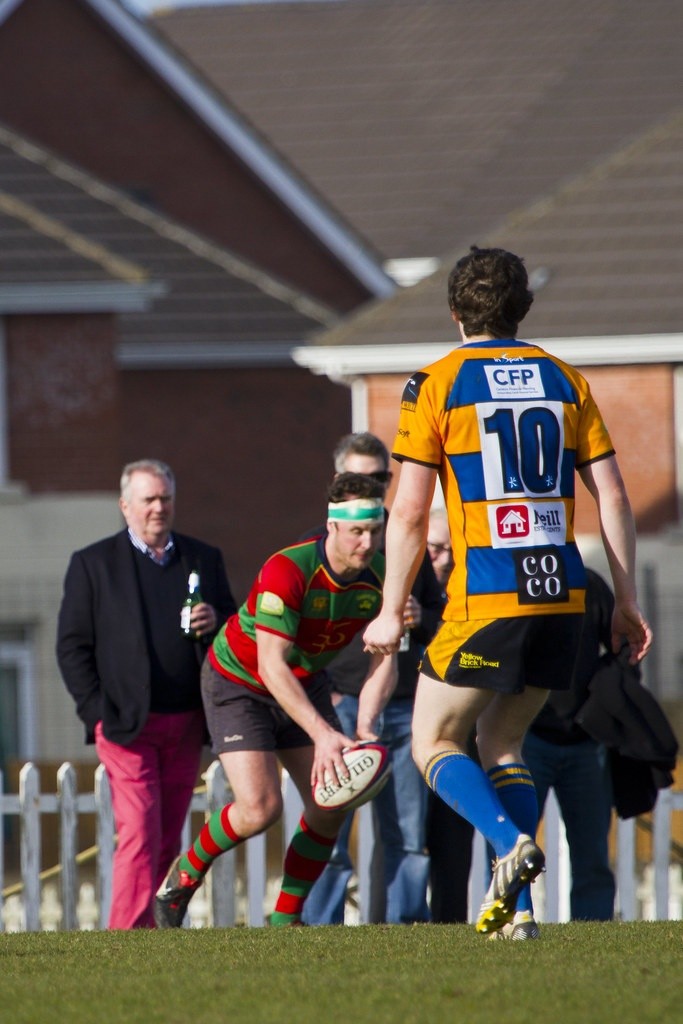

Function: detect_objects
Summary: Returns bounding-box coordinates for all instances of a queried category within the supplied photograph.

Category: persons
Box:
[363,249,655,940]
[153,432,641,930]
[56,459,239,930]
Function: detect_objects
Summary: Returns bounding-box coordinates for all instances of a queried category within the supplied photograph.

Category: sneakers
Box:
[264,914,305,928]
[486,909,538,941]
[152,854,203,929]
[476,834,546,933]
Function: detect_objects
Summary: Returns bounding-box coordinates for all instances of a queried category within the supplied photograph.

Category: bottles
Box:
[397,596,410,653]
[179,570,203,640]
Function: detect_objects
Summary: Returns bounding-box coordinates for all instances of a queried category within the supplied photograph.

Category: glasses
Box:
[427,541,452,554]
[368,471,390,484]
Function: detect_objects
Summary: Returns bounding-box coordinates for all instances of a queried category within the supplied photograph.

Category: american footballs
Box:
[311,738,394,813]
